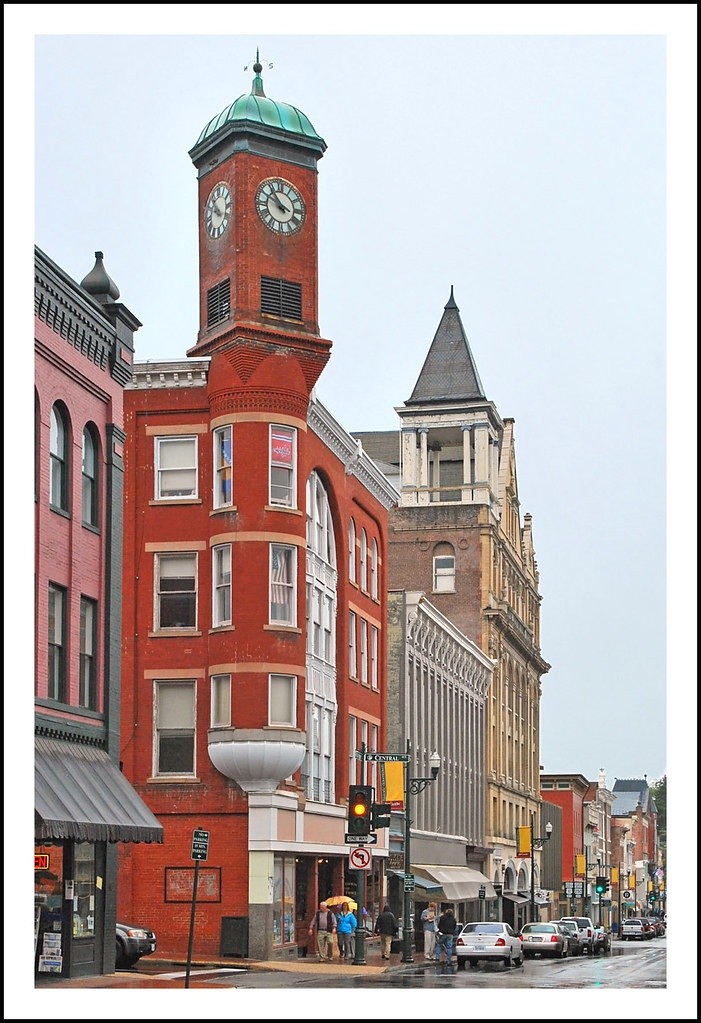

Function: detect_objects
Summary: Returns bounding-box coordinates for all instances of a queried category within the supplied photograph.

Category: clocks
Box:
[254,177,306,237]
[203,180,233,240]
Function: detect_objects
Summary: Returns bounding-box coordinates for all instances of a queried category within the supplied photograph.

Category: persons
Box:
[421,902,437,960]
[335,902,357,961]
[308,902,337,963]
[374,905,399,960]
[435,909,457,966]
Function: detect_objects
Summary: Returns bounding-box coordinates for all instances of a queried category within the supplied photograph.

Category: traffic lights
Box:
[650,891,658,902]
[596,876,609,893]
[372,803,392,828]
[348,784,372,833]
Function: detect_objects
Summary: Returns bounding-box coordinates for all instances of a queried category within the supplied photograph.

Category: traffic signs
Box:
[345,834,377,845]
[191,829,210,861]
[405,874,416,893]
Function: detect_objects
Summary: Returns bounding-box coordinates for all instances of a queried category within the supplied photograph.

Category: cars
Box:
[551,921,584,955]
[635,916,666,939]
[452,922,523,967]
[115,922,158,969]
[594,926,612,951]
[518,922,569,957]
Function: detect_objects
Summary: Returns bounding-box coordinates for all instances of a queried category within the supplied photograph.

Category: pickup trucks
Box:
[561,917,600,956]
[621,920,644,940]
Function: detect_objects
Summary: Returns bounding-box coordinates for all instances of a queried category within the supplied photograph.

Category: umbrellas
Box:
[325,896,355,906]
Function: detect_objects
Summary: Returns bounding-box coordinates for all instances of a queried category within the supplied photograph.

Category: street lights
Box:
[529,822,552,920]
[402,751,441,963]
[635,873,645,915]
[585,853,601,917]
[653,880,661,914]
[619,865,632,937]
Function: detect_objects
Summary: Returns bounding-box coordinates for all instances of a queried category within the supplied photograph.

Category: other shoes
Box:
[319,959,325,961]
[328,957,333,960]
[339,954,348,960]
[381,955,389,960]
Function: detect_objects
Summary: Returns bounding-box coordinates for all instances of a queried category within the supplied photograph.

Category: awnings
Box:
[520,893,550,908]
[34,735,165,847]
[504,894,530,909]
[410,864,497,904]
